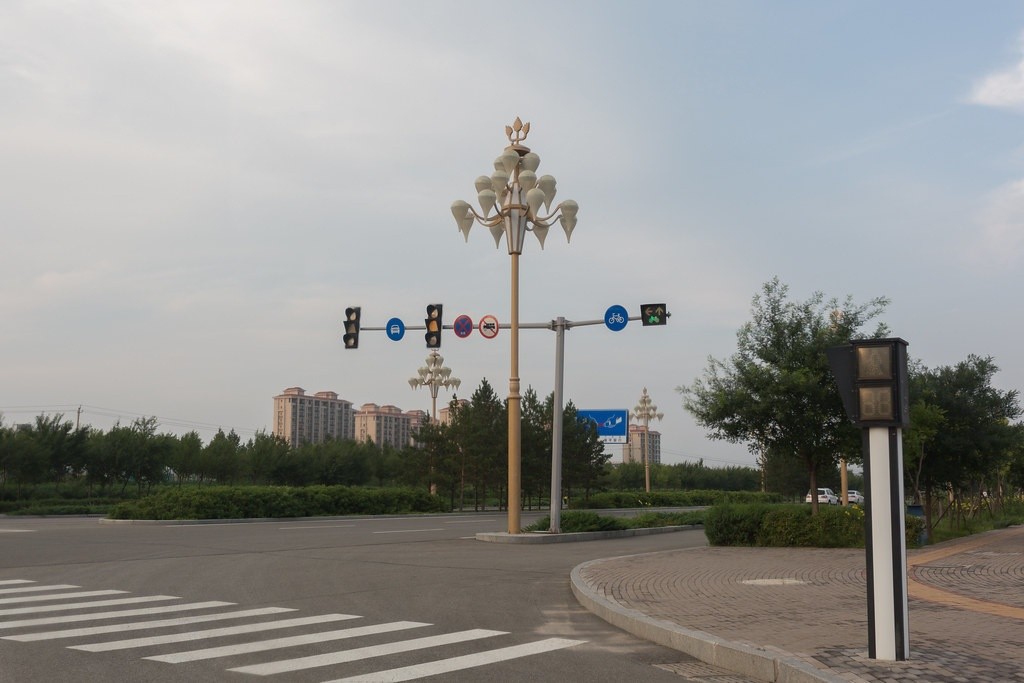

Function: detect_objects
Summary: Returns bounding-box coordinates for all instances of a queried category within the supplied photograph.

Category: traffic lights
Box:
[828,342,857,427]
[343,306,360,349]
[850,338,906,427]
[639,303,667,325]
[425,303,443,351]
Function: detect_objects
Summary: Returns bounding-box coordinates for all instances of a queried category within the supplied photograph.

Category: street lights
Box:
[451,118,578,535]
[407,352,463,497]
[628,388,665,493]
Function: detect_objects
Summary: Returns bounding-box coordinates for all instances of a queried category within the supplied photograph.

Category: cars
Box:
[840,490,865,504]
[806,488,839,507]
